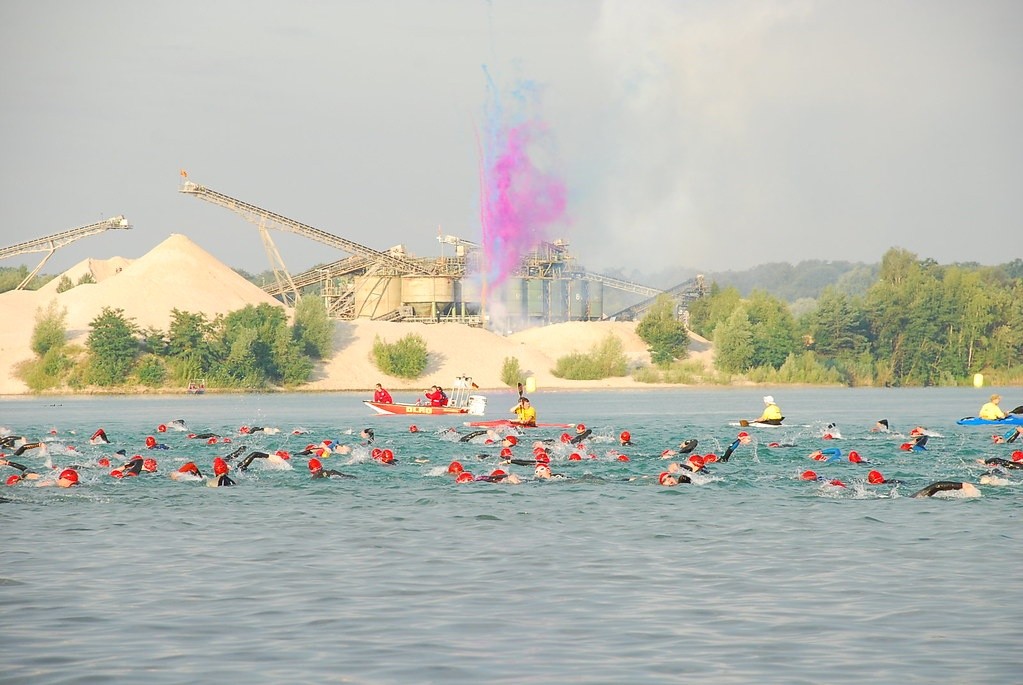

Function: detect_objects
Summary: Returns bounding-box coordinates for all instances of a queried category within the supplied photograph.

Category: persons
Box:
[0,420,398,488]
[992,426,1023,444]
[410,423,634,484]
[979,394,1010,420]
[374,384,393,404]
[979,451,1023,469]
[510,398,535,426]
[871,419,888,432]
[754,396,782,422]
[802,449,901,487]
[911,481,971,498]
[910,427,927,437]
[660,472,691,485]
[704,432,790,463]
[901,435,928,451]
[679,455,710,474]
[424,386,448,407]
[662,438,697,455]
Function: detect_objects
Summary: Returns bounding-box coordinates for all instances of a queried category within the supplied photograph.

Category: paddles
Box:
[740,420,758,427]
[518,382,523,421]
[1009,406,1023,414]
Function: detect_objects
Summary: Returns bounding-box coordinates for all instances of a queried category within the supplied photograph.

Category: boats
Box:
[957,414,1023,426]
[729,421,810,429]
[362,374,486,416]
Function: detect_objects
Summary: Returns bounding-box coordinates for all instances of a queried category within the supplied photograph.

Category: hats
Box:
[815,455,826,461]
[704,455,716,463]
[823,434,832,441]
[660,473,668,484]
[803,471,816,481]
[831,481,845,489]
[3,423,631,491]
[849,452,861,462]
[1012,451,1023,461]
[901,444,913,450]
[763,396,775,405]
[911,429,920,436]
[869,470,884,484]
[689,456,704,467]
[769,442,779,448]
[991,394,1002,401]
[738,432,747,438]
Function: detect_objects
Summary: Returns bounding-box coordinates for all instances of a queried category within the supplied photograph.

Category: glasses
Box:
[662,475,671,483]
[535,467,546,474]
[996,437,1002,442]
